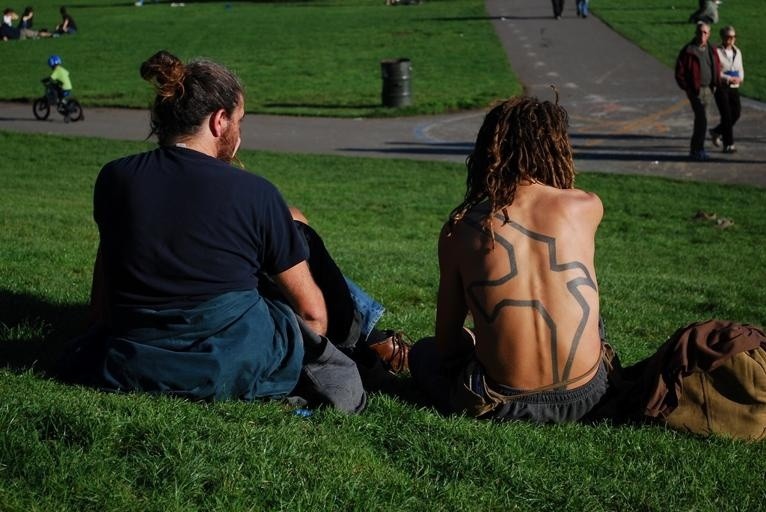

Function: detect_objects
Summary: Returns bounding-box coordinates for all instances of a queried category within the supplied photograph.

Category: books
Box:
[730,71,741,89]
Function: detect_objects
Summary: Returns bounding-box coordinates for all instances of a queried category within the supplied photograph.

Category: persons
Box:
[85,48,409,404]
[40,55,72,109]
[689,0,720,25]
[551,1,566,23]
[406,95,611,423]
[0,6,78,41]
[574,1,589,20]
[710,25,745,154]
[672,20,723,161]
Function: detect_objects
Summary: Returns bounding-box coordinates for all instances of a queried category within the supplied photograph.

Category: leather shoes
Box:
[370,329,413,372]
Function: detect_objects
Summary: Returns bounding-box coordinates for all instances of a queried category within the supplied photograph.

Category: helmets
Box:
[48,55,62,68]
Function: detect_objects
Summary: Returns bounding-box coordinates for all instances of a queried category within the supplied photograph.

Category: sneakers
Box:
[724,146,738,154]
[708,128,721,147]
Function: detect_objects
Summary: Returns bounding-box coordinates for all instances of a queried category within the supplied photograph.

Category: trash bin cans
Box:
[381,58,414,107]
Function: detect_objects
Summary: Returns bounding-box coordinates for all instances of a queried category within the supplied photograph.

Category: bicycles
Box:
[32,76,85,123]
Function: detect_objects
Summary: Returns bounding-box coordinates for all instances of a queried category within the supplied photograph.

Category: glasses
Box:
[724,33,737,40]
[697,31,709,35]
